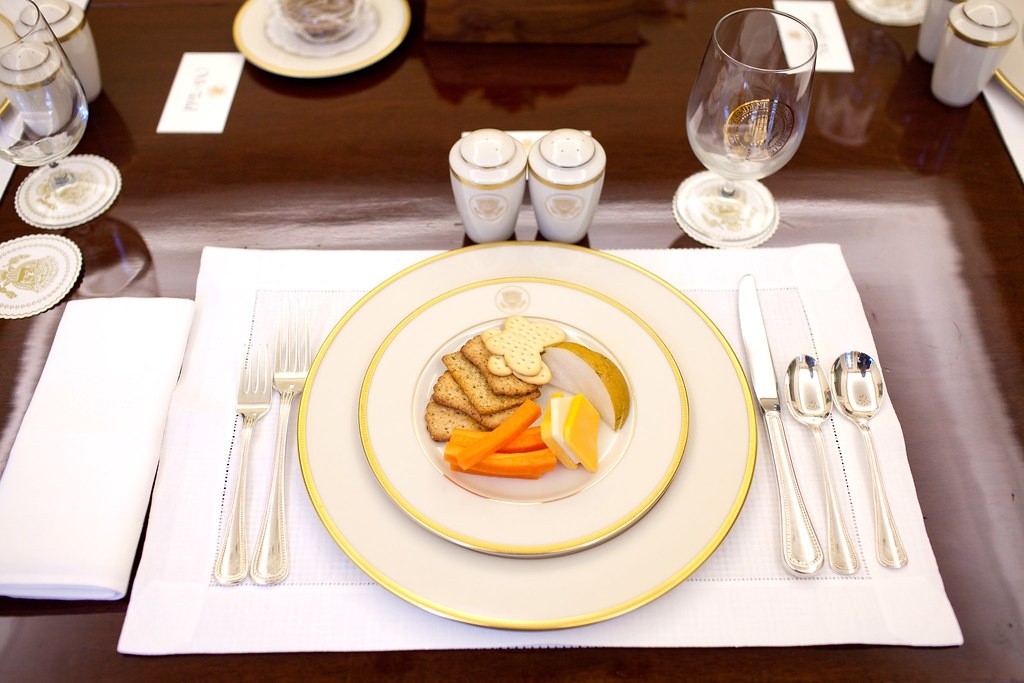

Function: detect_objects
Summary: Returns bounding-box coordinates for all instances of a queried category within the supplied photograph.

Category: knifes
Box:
[738,275,825,576]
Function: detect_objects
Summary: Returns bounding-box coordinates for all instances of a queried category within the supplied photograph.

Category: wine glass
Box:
[677,6,817,242]
[0,0,109,219]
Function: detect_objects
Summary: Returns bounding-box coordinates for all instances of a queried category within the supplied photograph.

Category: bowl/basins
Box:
[275,0,360,43]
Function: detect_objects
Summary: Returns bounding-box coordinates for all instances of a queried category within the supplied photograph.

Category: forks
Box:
[214,344,273,583]
[251,288,312,587]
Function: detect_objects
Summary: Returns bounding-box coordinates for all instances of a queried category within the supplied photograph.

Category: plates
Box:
[296,242,758,631]
[356,278,690,559]
[233,0,411,78]
[989,0,1024,106]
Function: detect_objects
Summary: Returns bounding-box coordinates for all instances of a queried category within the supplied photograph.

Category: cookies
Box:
[426,316,566,441]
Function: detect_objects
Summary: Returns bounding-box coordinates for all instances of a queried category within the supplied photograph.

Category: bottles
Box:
[447,127,528,244]
[917,0,969,61]
[13,0,102,104]
[528,128,607,245]
[931,0,1018,107]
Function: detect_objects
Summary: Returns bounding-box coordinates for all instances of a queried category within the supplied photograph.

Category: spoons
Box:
[784,353,861,574]
[829,351,909,569]
